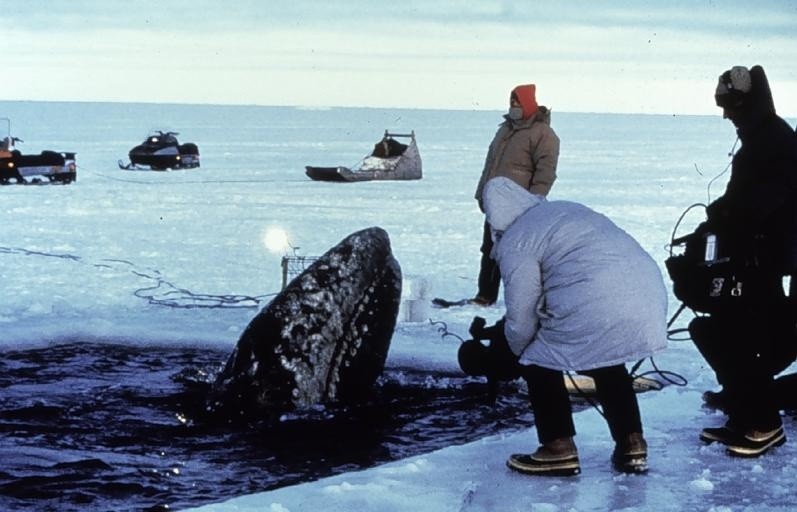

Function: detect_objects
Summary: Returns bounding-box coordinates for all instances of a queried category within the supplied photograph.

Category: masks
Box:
[509,106,524,120]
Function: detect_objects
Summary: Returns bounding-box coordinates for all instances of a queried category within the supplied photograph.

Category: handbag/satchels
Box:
[665,220,764,318]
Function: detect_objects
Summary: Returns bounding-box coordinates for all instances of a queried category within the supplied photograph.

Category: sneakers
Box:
[701,387,726,411]
[701,417,788,459]
[609,430,650,474]
[505,437,582,475]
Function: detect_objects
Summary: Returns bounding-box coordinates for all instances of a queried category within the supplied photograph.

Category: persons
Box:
[684,313,796,417]
[466,84,558,308]
[478,173,671,479]
[685,61,796,462]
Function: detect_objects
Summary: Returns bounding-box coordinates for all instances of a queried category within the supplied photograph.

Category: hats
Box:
[713,66,752,98]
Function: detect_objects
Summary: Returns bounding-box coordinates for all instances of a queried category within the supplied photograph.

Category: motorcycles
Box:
[0,134,77,186]
[116,131,200,171]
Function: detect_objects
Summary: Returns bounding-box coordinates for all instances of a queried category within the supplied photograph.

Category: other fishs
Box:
[206,223,404,444]
